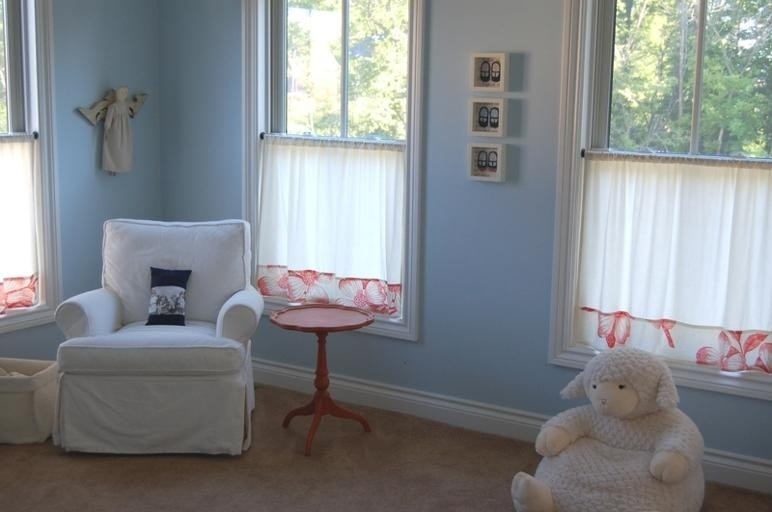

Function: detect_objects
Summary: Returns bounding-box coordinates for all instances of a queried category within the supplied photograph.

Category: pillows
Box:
[146,265,192,327]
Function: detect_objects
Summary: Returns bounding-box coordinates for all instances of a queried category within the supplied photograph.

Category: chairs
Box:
[53,216,265,456]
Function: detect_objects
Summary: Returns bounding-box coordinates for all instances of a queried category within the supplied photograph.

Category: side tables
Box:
[270,301,377,458]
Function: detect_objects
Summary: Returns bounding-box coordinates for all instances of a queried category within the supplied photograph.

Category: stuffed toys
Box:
[508,345,707,511]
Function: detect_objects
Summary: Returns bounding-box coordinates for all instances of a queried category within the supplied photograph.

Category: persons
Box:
[149,287,186,316]
[101,85,134,178]
